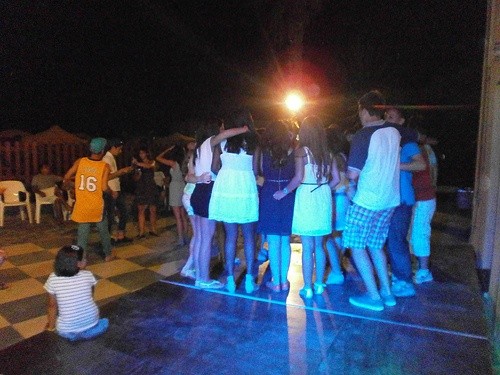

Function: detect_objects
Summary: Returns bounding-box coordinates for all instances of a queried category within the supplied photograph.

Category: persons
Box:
[64,138,118,262]
[32,108,436,298]
[131,148,165,240]
[345,90,437,311]
[44,244,109,341]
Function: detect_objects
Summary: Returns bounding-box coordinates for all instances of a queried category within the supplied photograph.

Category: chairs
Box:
[0,180,75,227]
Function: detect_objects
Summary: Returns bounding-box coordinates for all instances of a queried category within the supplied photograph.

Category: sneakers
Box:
[378,289,397,307]
[349,292,385,312]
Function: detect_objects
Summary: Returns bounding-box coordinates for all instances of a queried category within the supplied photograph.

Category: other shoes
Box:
[110,236,134,244]
[265,281,281,292]
[390,274,416,297]
[180,268,196,279]
[105,249,116,262]
[149,230,160,237]
[413,269,434,284]
[136,234,145,240]
[175,239,190,246]
[312,282,327,295]
[234,256,241,264]
[298,288,313,299]
[255,248,268,262]
[194,279,225,289]
[280,280,290,291]
[326,273,345,285]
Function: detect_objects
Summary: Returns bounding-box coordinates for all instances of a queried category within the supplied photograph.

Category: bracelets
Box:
[47,327,56,331]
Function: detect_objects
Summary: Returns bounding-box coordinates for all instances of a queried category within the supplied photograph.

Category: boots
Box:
[244,274,260,294]
[225,276,237,294]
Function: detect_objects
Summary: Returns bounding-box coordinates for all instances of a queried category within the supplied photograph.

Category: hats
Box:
[89,138,107,154]
[107,137,123,147]
